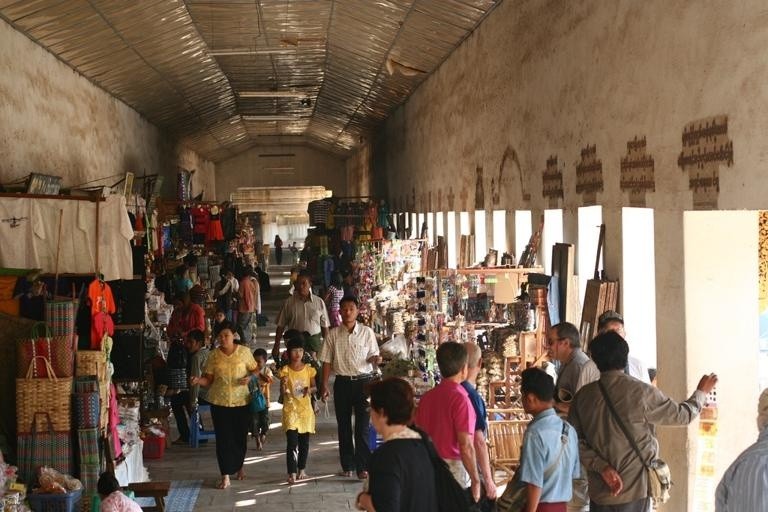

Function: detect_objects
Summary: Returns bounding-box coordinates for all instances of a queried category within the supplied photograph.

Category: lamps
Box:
[197,1,309,126]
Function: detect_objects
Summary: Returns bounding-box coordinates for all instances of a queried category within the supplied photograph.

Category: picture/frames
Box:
[121,171,136,207]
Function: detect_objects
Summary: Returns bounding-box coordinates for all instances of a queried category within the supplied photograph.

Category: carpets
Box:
[119,477,205,510]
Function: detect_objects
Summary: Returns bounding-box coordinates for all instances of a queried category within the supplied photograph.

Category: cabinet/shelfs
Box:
[440,265,546,400]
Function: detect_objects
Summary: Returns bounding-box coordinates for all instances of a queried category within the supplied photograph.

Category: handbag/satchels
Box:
[648,458,672,505]
[411,428,474,512]
[494,467,529,512]
[249,376,265,413]
[16,283,109,512]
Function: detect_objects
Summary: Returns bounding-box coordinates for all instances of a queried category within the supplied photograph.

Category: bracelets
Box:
[357,492,364,503]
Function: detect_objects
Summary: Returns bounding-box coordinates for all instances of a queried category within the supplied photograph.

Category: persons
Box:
[714,388,768,512]
[547,322,600,512]
[356,377,439,512]
[413,341,481,512]
[320,294,383,480]
[520,367,581,512]
[598,310,651,386]
[567,329,718,512]
[460,341,498,512]
[98,471,144,512]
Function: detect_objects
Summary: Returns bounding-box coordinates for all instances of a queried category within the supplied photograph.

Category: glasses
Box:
[547,338,562,345]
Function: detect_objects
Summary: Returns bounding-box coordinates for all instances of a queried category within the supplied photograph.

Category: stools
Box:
[187,400,218,450]
[124,479,173,512]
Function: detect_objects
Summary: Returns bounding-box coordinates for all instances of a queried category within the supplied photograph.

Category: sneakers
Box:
[171,438,190,445]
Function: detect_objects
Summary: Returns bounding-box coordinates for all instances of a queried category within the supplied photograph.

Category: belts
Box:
[336,374,374,382]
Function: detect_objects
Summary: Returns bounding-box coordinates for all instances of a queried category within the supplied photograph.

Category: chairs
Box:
[480,404,536,489]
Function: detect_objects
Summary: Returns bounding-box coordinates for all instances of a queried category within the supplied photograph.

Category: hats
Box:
[598,310,623,326]
